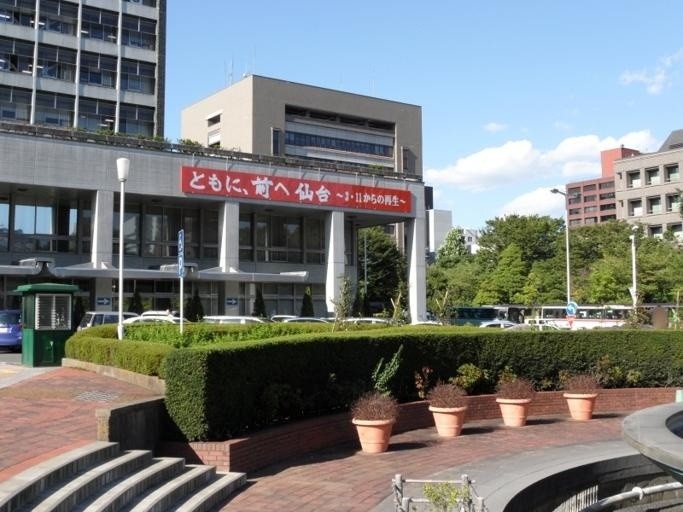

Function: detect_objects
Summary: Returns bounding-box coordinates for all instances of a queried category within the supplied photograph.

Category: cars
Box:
[123,310,443,332]
[0,308,22,353]
[476,318,570,331]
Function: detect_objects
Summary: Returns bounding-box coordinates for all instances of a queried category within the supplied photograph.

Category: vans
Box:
[78,311,138,335]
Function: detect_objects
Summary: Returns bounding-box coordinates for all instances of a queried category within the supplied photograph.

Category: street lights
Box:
[117,157,130,340]
[629,225,640,307]
[550,188,571,305]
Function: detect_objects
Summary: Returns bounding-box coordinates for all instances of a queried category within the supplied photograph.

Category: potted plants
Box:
[350,373,602,454]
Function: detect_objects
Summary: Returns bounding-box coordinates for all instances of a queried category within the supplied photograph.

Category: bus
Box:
[449,304,643,329]
